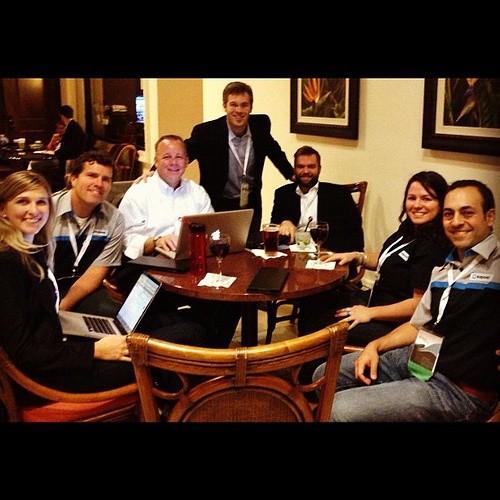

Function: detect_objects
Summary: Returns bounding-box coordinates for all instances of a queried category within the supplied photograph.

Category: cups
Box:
[262,224,281,257]
[296,224,311,248]
[17,138,26,149]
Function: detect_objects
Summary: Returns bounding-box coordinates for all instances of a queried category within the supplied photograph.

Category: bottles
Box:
[187,222,206,276]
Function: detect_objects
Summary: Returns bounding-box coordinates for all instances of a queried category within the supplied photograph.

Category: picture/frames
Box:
[289,78,360,142]
[419,78,500,165]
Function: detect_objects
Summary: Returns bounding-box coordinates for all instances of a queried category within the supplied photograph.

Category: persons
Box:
[135,82,295,244]
[311,180,500,423]
[50,151,125,312]
[46,105,85,176]
[299,171,450,400]
[270,147,364,287]
[118,134,215,259]
[1,170,178,421]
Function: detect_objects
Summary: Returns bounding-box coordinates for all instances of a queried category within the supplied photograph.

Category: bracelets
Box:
[153,235,161,246]
[357,252,366,265]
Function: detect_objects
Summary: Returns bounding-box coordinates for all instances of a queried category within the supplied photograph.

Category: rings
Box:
[347,312,350,315]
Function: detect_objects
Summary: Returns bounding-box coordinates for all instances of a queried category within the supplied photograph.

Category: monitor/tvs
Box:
[134,94,145,123]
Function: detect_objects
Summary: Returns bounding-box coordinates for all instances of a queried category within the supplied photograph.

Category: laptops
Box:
[58,272,161,337]
[156,209,254,260]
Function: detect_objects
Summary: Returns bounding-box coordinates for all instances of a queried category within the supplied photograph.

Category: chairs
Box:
[0,128,500,426]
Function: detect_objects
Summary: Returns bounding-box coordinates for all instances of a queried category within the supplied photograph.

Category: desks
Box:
[0,142,62,177]
[146,241,350,347]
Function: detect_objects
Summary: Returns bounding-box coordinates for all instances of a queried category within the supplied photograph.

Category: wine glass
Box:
[209,234,231,283]
[309,221,329,260]
